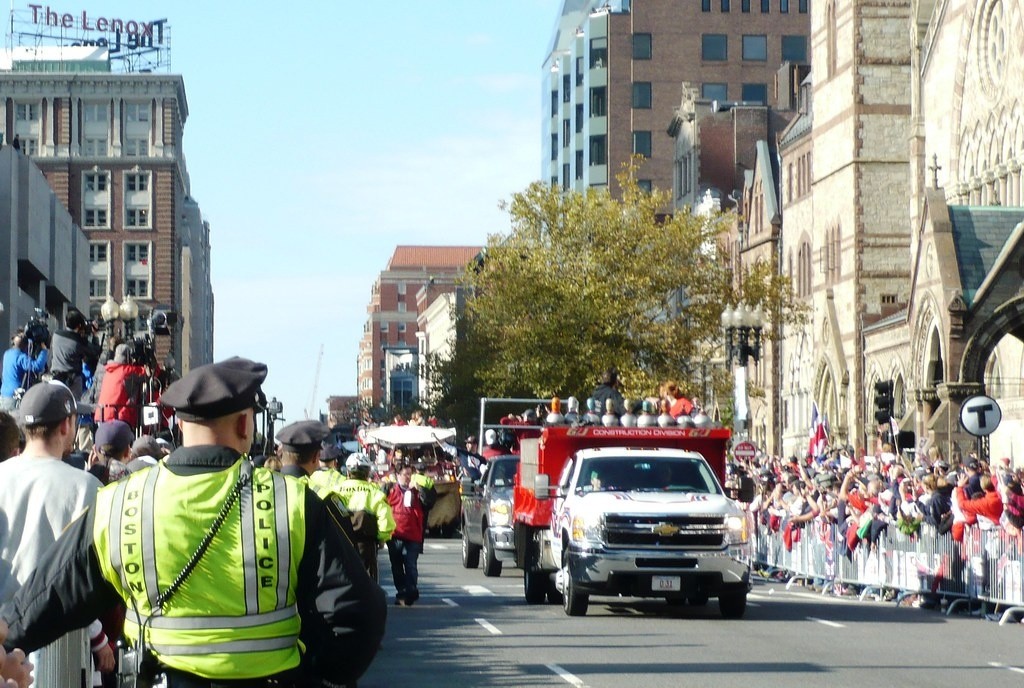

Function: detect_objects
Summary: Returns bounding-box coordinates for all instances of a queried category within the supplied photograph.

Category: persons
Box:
[0,309,697,688]
[724,437,1024,624]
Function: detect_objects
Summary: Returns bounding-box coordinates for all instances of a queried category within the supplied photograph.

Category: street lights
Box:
[723,302,766,436]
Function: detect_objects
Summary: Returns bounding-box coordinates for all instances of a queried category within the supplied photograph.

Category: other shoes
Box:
[759,571,1006,619]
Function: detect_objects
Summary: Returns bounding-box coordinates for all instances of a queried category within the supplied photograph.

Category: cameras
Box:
[82,319,94,335]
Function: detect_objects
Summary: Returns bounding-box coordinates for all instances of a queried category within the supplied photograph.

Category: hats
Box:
[964,456,980,470]
[20,341,164,462]
[465,437,480,446]
[1003,458,1011,466]
[485,429,496,445]
[345,452,371,476]
[158,355,268,422]
[253,420,340,469]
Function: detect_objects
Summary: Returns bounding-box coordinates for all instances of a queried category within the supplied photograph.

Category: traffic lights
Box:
[873,379,893,426]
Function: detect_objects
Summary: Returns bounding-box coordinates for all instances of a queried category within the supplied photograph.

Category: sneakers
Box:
[394,587,419,606]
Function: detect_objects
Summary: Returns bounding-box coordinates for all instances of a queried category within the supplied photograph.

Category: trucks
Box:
[460,454,525,578]
[511,419,756,618]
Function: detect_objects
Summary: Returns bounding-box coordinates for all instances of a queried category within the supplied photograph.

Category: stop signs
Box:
[733,442,755,463]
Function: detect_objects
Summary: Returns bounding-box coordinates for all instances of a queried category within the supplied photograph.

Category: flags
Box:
[808,403,830,462]
[890,418,898,435]
